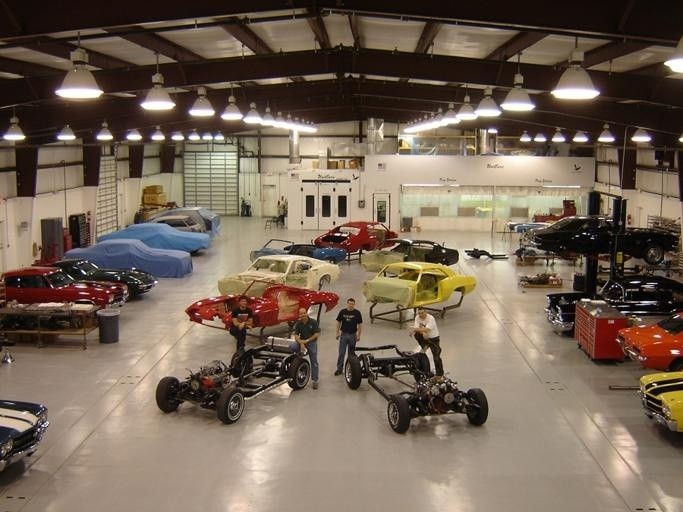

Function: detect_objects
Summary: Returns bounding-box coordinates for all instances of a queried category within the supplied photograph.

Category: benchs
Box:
[265,220,280,231]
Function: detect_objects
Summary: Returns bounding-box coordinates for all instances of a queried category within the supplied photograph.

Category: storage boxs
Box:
[313,160,345,169]
[349,160,358,169]
[143,185,166,210]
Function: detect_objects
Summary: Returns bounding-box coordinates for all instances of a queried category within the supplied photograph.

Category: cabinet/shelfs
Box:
[646,214,683,267]
[0,278,101,350]
[397,119,478,157]
[495,134,536,154]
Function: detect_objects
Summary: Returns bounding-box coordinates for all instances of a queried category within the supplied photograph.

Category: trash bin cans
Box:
[241,205,252,216]
[96,308,121,344]
[403,217,413,231]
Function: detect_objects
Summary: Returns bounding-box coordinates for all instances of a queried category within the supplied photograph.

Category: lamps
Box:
[2,106,27,141]
[663,34,683,73]
[54,30,320,141]
[402,37,653,143]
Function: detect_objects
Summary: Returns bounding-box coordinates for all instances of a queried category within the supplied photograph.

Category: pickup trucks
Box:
[527,214,678,265]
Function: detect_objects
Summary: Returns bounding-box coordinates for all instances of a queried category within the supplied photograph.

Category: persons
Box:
[334,298,363,376]
[245,193,253,216]
[241,196,245,216]
[409,305,444,376]
[291,306,320,389]
[229,296,253,351]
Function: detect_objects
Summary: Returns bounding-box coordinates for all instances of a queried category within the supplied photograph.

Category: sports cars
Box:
[638,368,682,435]
[617,311,682,371]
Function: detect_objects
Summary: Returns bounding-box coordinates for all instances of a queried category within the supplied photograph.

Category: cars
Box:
[0,206,221,313]
[545,273,681,338]
[0,399,50,472]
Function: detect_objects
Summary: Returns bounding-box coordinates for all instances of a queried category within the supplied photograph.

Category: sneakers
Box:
[312,381,319,390]
[334,368,343,376]
